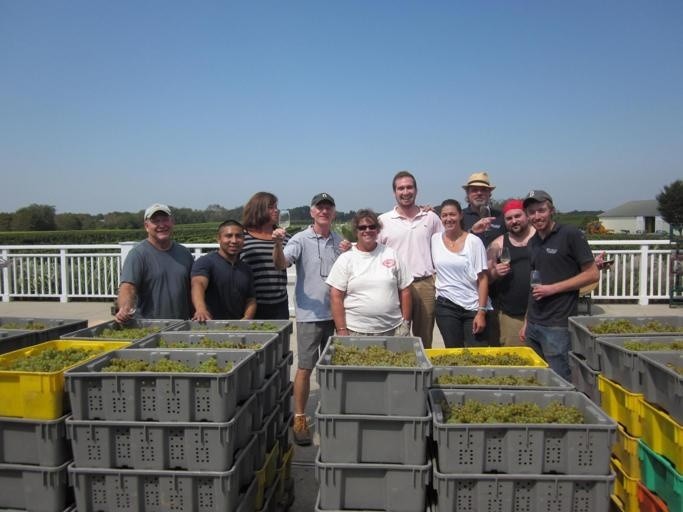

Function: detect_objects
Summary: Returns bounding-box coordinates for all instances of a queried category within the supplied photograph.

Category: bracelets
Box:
[477,305,490,314]
[333,325,347,333]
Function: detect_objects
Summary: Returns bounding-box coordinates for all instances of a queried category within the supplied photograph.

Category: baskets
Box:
[567,317,683,510]
[314,336,616,511]
[1,316,294,511]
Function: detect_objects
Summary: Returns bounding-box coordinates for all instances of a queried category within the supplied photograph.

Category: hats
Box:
[144,204,171,220]
[462,172,495,191]
[311,193,335,207]
[523,191,552,208]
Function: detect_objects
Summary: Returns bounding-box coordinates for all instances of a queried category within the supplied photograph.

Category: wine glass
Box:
[119,295,137,320]
[479,205,491,236]
[499,245,511,275]
[531,271,542,301]
[279,210,291,240]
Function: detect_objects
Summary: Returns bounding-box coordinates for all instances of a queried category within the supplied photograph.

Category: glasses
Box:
[357,225,376,230]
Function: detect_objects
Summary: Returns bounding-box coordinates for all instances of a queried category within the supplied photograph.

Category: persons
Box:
[337,171,448,349]
[240,190,294,322]
[482,199,615,347]
[117,204,197,329]
[325,210,414,340]
[516,187,603,385]
[428,199,490,349]
[268,190,348,449]
[189,219,261,325]
[453,169,510,345]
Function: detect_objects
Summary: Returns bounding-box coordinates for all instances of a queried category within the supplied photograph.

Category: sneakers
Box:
[293,414,311,446]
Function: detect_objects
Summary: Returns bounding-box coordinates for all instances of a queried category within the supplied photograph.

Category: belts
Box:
[414,277,429,282]
